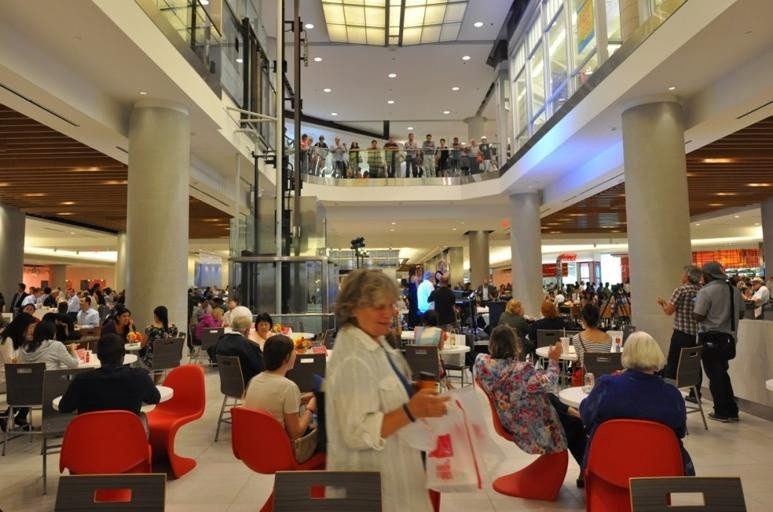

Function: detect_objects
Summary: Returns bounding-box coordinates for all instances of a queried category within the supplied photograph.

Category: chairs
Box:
[59,410,153,503]
[583,420,684,512]
[230,408,326,512]
[273,470,382,512]
[145,365,205,480]
[628,476,747,512]
[476,378,568,502]
[55,473,166,512]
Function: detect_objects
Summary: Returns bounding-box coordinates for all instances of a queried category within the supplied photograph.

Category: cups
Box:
[615,336,620,345]
[449,335,455,347]
[84,350,93,364]
[560,338,570,354]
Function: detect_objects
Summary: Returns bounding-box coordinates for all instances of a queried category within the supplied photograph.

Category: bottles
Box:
[585,372,595,386]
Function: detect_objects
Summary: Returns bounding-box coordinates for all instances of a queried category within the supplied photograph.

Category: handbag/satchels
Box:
[729,336,735,360]
[442,328,467,367]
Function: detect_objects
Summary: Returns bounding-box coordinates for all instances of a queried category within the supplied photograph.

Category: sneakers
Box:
[708,412,739,422]
[685,395,698,403]
[14,416,34,431]
[0,412,9,431]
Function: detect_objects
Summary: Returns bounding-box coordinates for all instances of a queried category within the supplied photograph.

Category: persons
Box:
[1,261,319,489]
[284,128,511,178]
[579,331,696,476]
[402,263,772,423]
[474,323,590,483]
[326,268,451,512]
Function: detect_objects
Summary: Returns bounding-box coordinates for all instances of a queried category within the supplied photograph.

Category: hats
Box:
[703,262,728,279]
[751,277,764,283]
[737,280,748,290]
